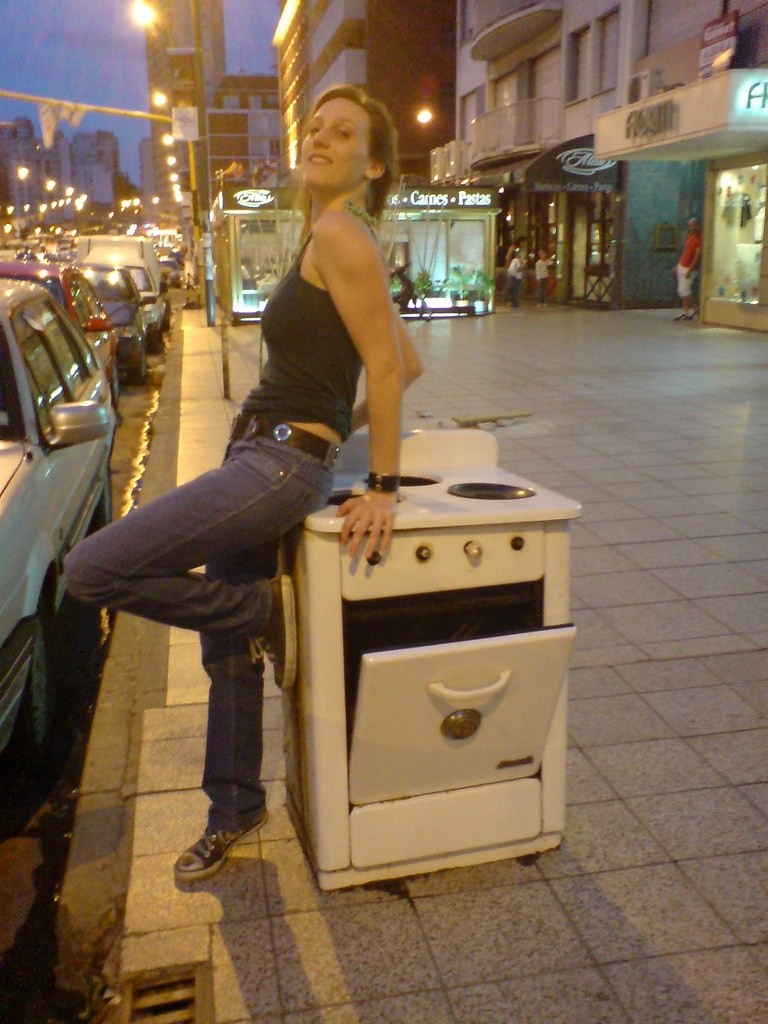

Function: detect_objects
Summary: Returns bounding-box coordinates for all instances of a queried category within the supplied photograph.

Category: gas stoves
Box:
[276,429,582,530]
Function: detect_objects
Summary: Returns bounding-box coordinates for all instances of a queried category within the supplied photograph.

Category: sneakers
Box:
[248,575,297,691]
[174,808,269,884]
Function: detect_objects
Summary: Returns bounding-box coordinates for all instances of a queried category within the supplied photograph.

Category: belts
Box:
[232,416,340,462]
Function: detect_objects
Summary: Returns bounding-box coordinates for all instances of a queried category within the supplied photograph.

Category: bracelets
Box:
[368,472,400,492]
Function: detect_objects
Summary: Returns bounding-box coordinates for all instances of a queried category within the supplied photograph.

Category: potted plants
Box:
[440,264,496,306]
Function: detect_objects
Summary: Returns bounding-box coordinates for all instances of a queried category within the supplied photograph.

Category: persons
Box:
[536,249,554,307]
[672,218,701,320]
[65,85,424,881]
[505,236,528,310]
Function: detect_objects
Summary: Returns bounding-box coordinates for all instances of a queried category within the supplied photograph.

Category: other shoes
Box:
[674,311,699,320]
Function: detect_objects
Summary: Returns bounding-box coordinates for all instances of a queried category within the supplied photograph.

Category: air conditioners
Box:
[626,67,666,104]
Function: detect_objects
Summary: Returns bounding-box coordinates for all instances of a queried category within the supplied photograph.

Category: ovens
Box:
[270,520,578,888]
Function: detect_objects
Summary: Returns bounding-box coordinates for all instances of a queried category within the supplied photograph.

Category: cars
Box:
[5,231,187,355]
[1,278,117,760]
[1,262,120,411]
[66,261,158,385]
